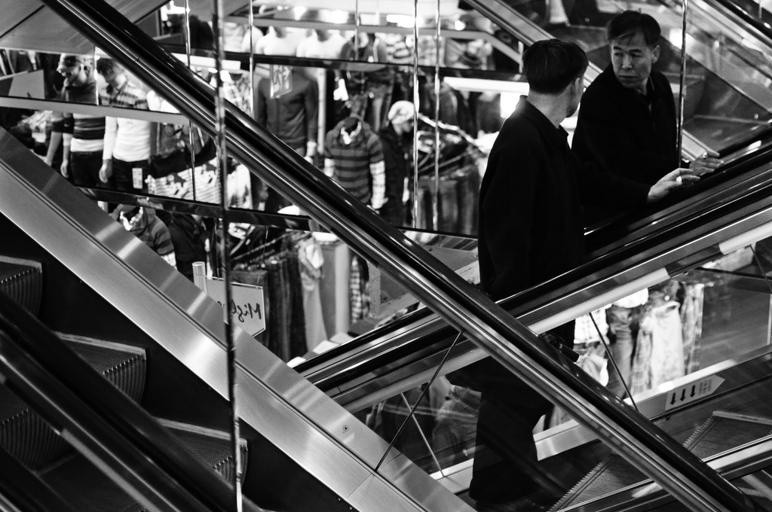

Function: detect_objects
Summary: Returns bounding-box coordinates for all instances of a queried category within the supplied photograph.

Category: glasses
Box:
[403,118,419,125]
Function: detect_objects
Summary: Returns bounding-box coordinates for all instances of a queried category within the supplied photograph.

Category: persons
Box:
[470,41,699,512]
[574,10,722,403]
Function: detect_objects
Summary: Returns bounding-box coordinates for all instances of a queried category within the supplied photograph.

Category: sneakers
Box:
[511,463,569,504]
[474,493,550,512]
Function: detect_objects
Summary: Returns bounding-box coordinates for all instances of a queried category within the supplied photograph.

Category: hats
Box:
[384,99,415,125]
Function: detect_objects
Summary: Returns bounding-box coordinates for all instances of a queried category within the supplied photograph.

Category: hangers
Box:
[231,234,292,272]
